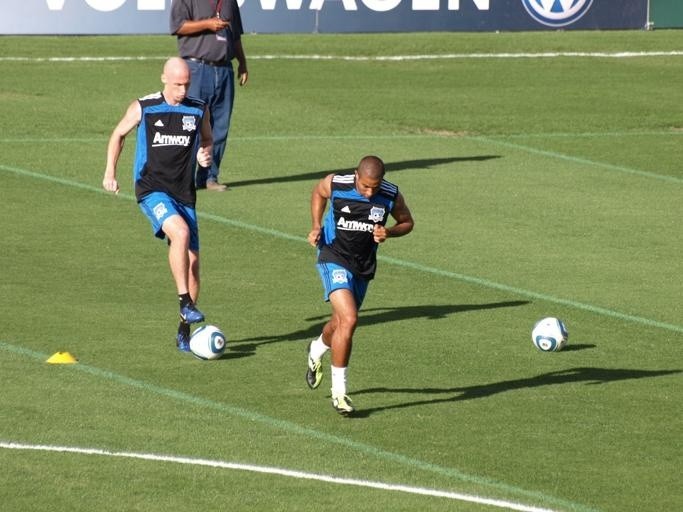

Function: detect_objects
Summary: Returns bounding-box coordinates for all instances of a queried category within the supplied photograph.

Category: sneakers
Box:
[301,339,324,390]
[178,302,204,325]
[205,180,228,191]
[174,332,192,354]
[329,393,356,415]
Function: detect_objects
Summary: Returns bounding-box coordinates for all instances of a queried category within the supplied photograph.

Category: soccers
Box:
[189,326,225,360]
[531,317,568,352]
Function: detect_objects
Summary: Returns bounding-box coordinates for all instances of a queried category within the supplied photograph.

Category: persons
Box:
[169,1,248,193]
[305,155,416,415]
[102,57,213,352]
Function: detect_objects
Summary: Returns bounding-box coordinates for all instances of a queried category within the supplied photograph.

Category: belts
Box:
[182,57,230,67]
